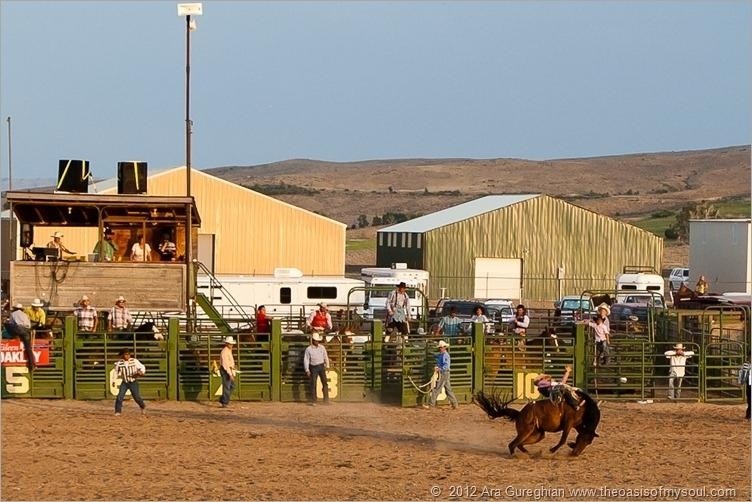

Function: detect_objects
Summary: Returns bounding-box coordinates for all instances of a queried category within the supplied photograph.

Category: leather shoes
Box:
[574,400,586,411]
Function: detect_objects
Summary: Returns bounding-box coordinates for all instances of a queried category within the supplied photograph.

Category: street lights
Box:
[175,0,206,325]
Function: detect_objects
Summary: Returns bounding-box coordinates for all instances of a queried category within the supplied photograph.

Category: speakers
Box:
[59,160,89,192]
[20,222,33,248]
[118,162,147,194]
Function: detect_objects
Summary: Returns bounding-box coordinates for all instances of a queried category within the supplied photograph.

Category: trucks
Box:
[124,266,370,351]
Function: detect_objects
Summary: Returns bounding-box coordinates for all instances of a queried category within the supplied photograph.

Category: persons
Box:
[129,234,152,262]
[46,232,78,261]
[157,233,173,261]
[9,275,752,424]
[92,227,121,263]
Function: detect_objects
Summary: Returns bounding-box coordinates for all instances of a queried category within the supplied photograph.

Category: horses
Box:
[128,321,164,358]
[527,324,561,368]
[472,380,601,459]
[329,327,349,367]
[196,328,258,370]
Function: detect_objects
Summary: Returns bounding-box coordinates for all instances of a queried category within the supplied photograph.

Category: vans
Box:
[613,265,665,302]
[362,262,428,321]
[668,268,690,291]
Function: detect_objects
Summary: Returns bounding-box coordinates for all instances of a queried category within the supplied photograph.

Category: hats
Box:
[436,340,450,348]
[223,336,236,345]
[629,316,638,321]
[593,315,605,323]
[312,333,323,341]
[317,302,329,311]
[533,372,550,387]
[673,343,686,350]
[396,282,409,289]
[50,229,172,243]
[597,305,611,317]
[12,296,126,310]
[119,348,133,355]
[473,305,485,315]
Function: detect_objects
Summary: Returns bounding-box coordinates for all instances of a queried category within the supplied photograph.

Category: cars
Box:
[429,292,751,373]
